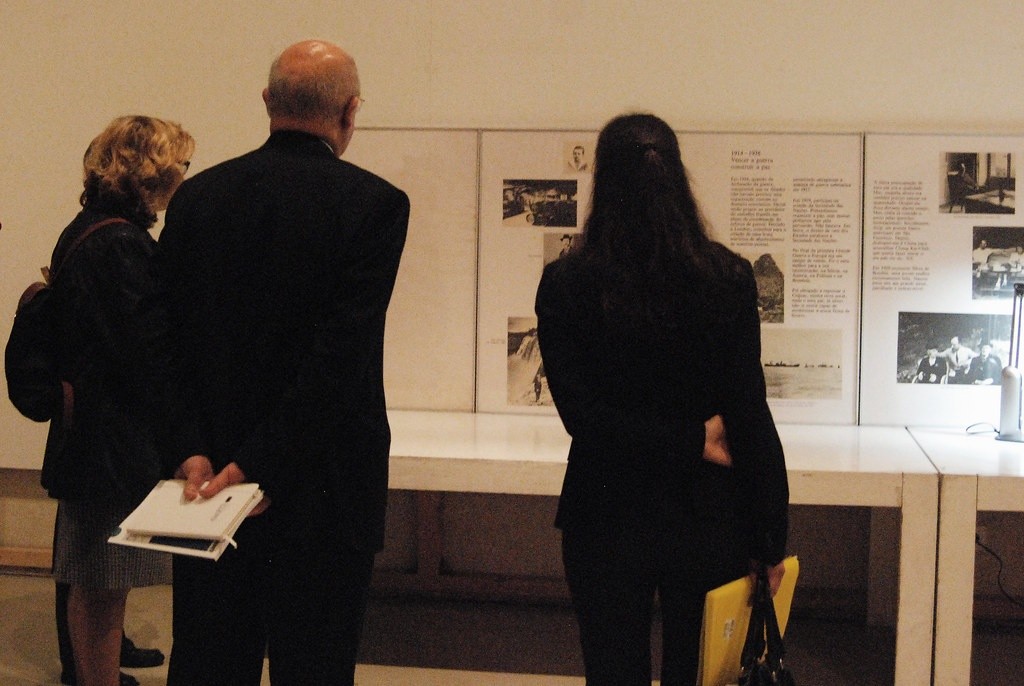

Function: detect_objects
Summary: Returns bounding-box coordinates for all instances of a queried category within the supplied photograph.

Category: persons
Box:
[972,239,1024,290]
[559,234,574,258]
[147,39,411,686]
[39,114,195,686]
[535,112,790,686]
[916,335,1002,385]
[953,160,988,205]
[568,146,588,174]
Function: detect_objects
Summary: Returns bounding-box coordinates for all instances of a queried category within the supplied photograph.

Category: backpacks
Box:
[5,218,132,422]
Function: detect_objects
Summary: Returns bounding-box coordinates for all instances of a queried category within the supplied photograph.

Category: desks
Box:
[908,424,1024,686]
[386,409,940,686]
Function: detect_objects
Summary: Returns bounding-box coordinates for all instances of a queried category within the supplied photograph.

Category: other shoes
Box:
[119,671,140,686]
[119,638,164,667]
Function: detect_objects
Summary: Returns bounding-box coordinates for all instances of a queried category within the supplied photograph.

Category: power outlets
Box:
[975,525,988,552]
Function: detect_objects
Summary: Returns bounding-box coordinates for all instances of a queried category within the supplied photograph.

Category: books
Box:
[107,478,264,561]
